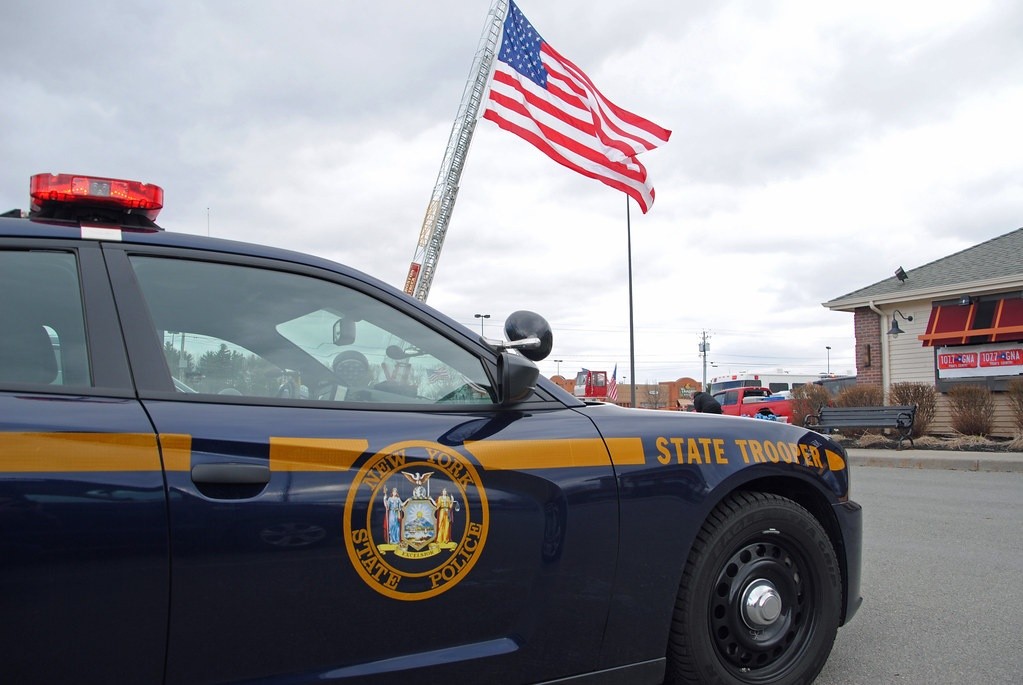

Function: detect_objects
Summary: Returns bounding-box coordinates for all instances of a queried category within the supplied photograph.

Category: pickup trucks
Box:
[688,386,824,423]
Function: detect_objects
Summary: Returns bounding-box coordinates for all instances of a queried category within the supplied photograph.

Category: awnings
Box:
[917,289,1023,347]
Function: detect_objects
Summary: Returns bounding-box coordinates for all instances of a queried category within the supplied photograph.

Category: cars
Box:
[0,171,866,685]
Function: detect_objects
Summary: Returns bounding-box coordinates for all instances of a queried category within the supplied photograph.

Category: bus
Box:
[710,375,847,395]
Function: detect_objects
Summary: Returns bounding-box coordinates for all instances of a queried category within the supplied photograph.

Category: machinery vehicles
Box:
[404,0,608,402]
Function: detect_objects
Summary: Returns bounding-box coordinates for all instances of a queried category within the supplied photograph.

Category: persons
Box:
[690,390,722,414]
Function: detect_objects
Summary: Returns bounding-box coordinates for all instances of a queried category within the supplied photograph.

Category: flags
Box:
[426,368,449,383]
[481,0,673,215]
[607,366,617,401]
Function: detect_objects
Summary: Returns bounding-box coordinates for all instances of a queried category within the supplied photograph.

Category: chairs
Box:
[0,318,57,382]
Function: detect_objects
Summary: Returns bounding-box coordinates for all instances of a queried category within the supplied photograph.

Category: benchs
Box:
[802,405,918,451]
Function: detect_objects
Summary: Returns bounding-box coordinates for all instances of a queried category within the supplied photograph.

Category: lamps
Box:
[895,266,908,282]
[958,295,980,307]
[886,310,913,339]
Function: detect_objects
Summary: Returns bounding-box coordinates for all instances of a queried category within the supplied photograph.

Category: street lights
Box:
[474,314,490,336]
[826,347,831,374]
[623,377,626,382]
[553,360,562,376]
